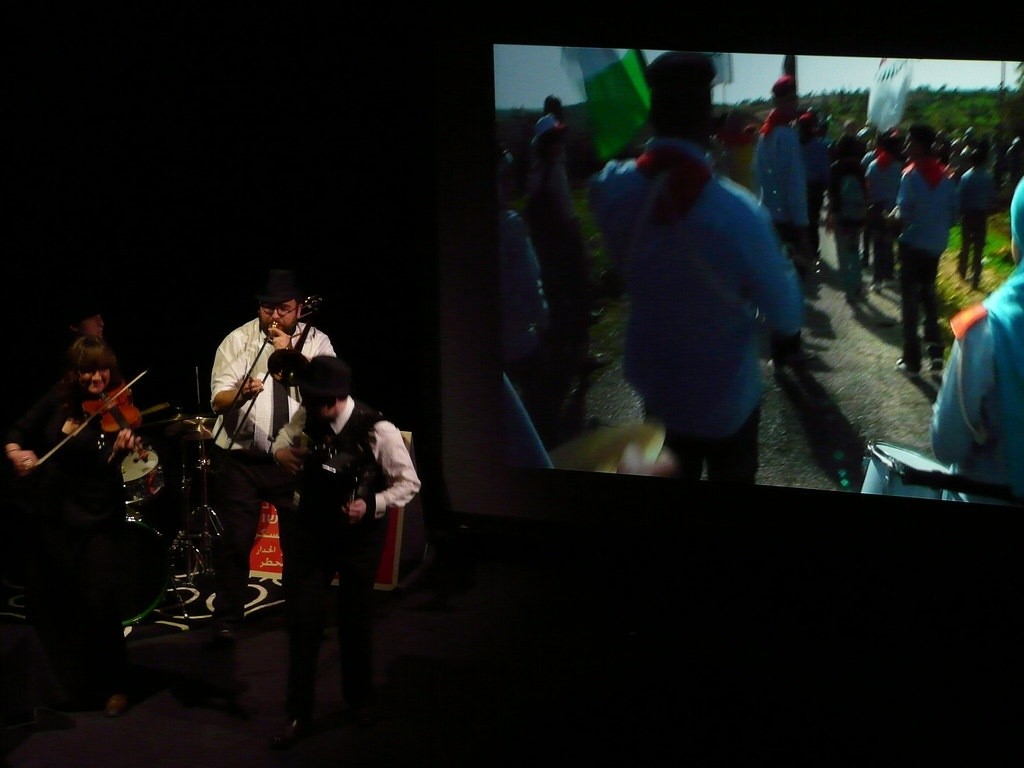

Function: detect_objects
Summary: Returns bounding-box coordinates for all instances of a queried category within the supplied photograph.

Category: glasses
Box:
[262,305,298,318]
[255,302,298,318]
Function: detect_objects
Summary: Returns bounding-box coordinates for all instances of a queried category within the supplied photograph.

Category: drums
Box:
[120,505,171,626]
[855,439,948,499]
[121,447,165,505]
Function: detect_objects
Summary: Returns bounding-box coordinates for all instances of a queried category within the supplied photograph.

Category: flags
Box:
[560,46,651,160]
[865,56,914,133]
[710,53,733,88]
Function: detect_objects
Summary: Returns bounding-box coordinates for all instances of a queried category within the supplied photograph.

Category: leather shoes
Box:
[105,693,128,717]
[353,706,377,729]
[269,719,314,749]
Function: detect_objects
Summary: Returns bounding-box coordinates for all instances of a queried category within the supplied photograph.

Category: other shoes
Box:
[211,622,237,642]
[929,344,947,373]
[899,358,923,379]
[769,330,817,363]
[957,267,967,281]
[966,272,982,289]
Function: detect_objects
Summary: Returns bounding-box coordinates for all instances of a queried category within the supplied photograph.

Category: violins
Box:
[80,379,153,464]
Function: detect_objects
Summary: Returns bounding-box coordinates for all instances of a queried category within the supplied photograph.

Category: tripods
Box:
[168,438,225,588]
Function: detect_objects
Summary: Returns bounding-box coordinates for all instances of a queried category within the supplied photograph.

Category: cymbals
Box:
[150,411,213,426]
[167,416,217,441]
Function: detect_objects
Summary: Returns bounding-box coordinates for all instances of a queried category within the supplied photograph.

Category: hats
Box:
[645,52,716,96]
[773,78,795,98]
[544,97,563,114]
[299,356,352,395]
[255,270,305,304]
[535,113,561,143]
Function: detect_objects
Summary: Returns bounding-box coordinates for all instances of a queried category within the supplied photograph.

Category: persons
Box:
[441,53,1024,519]
[4,269,420,746]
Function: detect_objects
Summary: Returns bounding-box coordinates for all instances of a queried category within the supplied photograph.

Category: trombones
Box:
[196,288,327,473]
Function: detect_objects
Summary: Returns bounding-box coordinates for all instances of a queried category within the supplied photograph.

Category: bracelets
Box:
[6,450,16,454]
